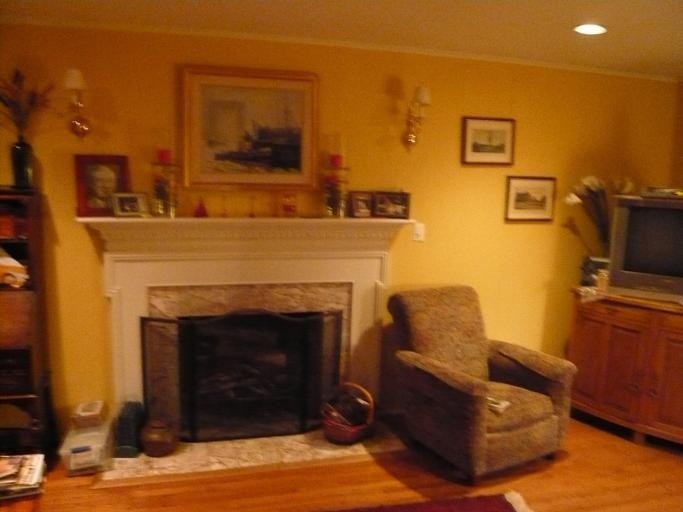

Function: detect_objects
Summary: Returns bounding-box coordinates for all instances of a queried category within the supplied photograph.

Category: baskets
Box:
[324,382,378,445]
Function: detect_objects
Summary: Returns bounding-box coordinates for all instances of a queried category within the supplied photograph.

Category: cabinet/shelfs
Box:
[0,190,43,456]
[559,284,683,450]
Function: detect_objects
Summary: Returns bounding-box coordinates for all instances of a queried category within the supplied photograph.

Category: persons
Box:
[357,196,371,213]
[85,165,118,207]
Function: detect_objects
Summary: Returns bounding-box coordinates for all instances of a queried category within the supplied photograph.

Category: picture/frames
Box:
[506,176,557,222]
[460,116,516,164]
[348,190,374,218]
[179,63,317,192]
[73,154,131,218]
[110,192,149,216]
[372,190,412,217]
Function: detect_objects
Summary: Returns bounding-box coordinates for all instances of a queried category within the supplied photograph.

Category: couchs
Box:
[387,286,578,487]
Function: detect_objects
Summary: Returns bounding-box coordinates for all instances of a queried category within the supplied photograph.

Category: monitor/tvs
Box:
[608,193,683,306]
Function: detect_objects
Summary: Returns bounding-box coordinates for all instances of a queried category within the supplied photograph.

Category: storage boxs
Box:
[58,423,119,476]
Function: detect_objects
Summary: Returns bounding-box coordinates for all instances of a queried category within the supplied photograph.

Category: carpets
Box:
[343,489,534,512]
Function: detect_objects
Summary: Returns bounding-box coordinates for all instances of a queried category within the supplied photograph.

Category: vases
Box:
[12,135,32,190]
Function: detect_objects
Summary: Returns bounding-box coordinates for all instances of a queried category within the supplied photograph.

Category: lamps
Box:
[59,68,90,137]
[407,84,430,143]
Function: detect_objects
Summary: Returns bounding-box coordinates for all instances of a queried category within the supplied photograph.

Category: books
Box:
[1,454,47,500]
[487,395,510,413]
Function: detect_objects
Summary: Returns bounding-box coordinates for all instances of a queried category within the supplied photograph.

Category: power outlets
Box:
[413,223,428,242]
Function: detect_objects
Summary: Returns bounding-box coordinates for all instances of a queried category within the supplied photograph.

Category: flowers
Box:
[0,66,55,134]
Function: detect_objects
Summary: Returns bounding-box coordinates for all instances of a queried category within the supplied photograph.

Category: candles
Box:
[155,145,171,165]
[328,154,344,168]
